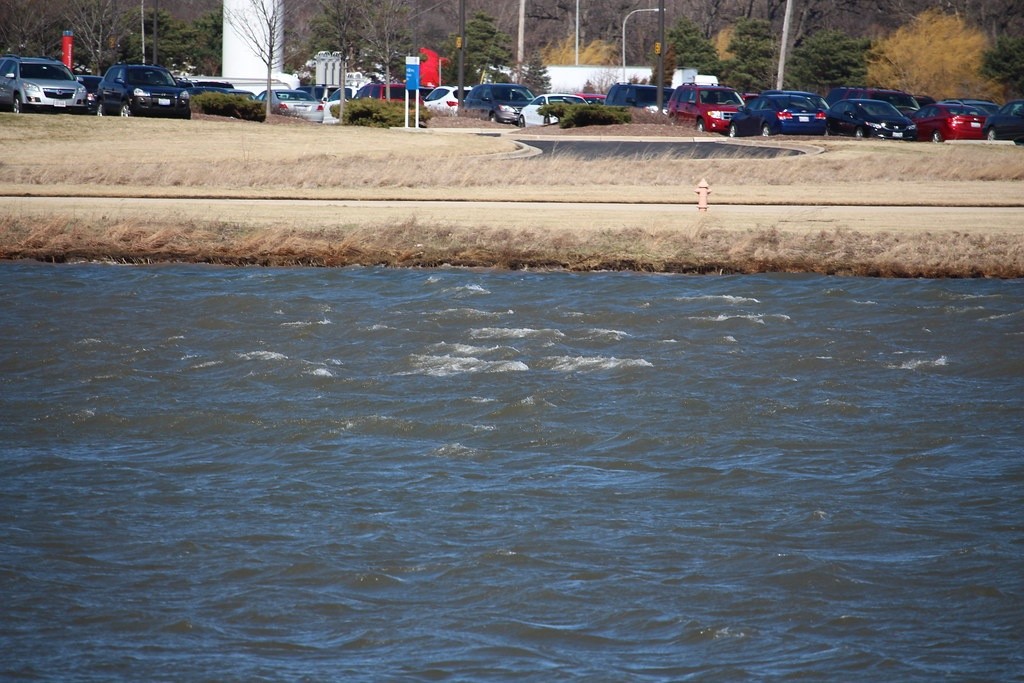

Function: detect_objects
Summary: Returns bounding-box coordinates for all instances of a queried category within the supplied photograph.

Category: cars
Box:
[729,95,827,138]
[177,81,360,124]
[353,83,424,106]
[742,89,829,112]
[420,86,472,116]
[911,103,991,143]
[74,75,103,115]
[518,94,589,127]
[982,99,1024,146]
[576,82,675,107]
[826,98,918,139]
[827,86,1002,120]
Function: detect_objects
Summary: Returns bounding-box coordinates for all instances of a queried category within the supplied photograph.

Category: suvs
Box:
[0,54,89,115]
[667,81,746,132]
[461,84,543,124]
[96,61,190,119]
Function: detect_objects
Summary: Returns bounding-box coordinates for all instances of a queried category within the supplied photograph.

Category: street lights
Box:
[622,8,666,83]
